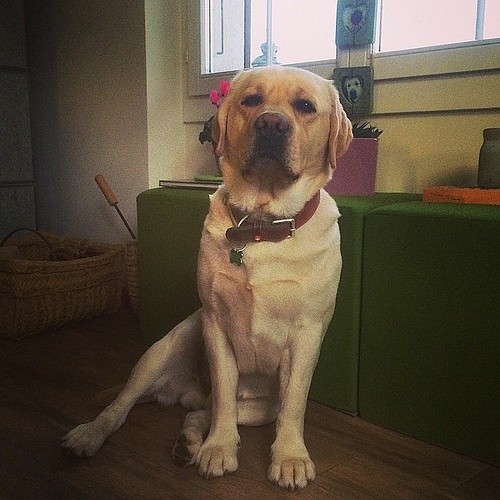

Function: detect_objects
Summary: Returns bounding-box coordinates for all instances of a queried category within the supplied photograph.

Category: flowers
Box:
[199,79,231,145]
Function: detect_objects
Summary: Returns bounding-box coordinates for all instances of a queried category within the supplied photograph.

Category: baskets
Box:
[0,230,128,341]
[124,239,140,317]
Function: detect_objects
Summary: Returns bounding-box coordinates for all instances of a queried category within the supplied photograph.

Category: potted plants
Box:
[323,121,384,197]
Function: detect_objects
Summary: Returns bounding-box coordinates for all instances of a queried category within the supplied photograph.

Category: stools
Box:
[136,187,500,471]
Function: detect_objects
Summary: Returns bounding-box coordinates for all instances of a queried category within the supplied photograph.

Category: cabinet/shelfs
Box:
[0,0,36,245]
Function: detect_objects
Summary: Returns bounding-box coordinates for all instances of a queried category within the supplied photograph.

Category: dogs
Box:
[57,65,355,490]
